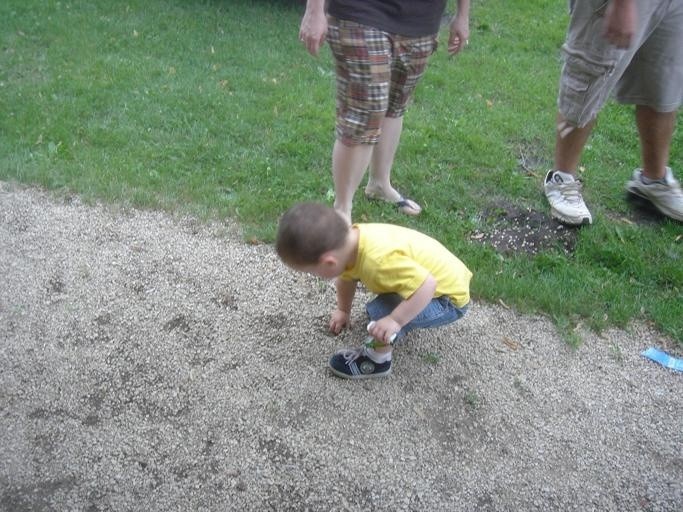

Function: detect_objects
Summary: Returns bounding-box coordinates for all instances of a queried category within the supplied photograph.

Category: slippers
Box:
[367,195,422,216]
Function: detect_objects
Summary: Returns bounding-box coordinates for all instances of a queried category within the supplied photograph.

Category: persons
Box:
[274,200,474,383]
[545,0,683,229]
[296,0,475,230]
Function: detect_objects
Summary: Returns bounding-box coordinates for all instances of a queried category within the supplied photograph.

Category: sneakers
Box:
[329,345,392,379]
[543,169,593,226]
[624,166,683,223]
